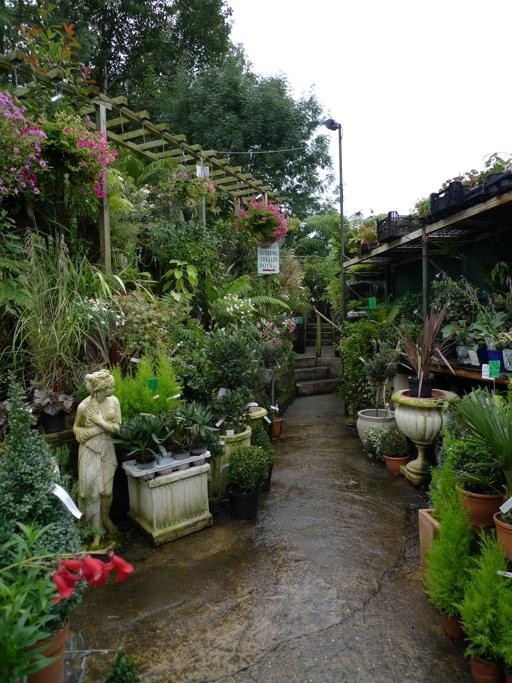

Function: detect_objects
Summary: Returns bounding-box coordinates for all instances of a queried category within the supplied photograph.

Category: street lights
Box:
[324,119,347,323]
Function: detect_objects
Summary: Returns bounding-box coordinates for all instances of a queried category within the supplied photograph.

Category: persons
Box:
[73,369,122,549]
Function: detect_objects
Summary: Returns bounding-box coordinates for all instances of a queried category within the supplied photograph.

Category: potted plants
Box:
[114,328,292,548]
[348,300,512,487]
[0,518,95,681]
[418,386,512,680]
